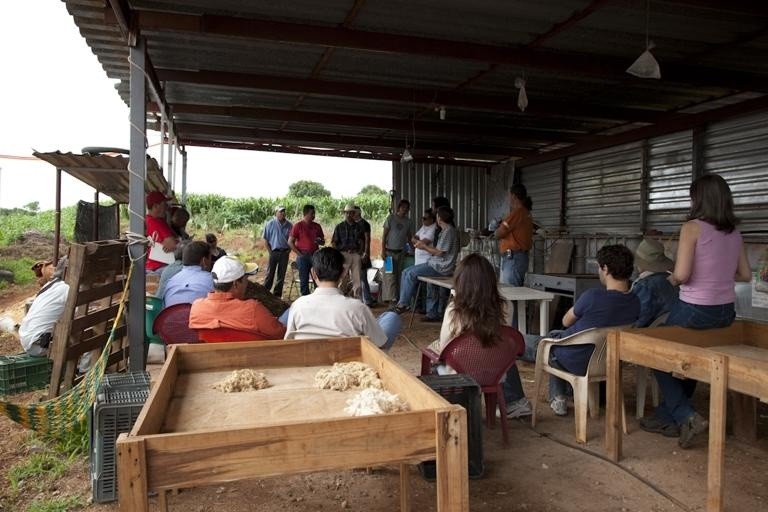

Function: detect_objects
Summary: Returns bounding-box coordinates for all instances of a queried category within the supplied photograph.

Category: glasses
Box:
[421,216,433,221]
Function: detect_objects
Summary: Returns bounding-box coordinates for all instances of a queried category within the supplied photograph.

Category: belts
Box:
[512,248,530,254]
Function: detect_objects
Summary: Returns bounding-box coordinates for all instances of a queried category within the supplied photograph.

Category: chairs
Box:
[633,310,669,421]
[418,325,526,446]
[145,296,169,345]
[199,325,265,342]
[288,261,318,304]
[151,301,205,342]
[531,319,628,443]
[282,329,345,338]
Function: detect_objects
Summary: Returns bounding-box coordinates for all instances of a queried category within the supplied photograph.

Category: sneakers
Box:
[677,412,709,450]
[549,395,569,417]
[496,401,532,419]
[638,414,680,438]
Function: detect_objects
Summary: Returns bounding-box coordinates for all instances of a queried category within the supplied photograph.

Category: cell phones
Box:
[664,271,672,277]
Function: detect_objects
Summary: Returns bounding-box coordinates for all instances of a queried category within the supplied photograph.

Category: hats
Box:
[211,255,259,285]
[146,191,173,209]
[274,203,288,212]
[338,204,361,213]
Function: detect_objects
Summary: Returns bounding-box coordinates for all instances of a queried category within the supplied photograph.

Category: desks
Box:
[408,273,515,334]
[603,318,767,512]
[448,282,555,348]
[115,333,472,512]
